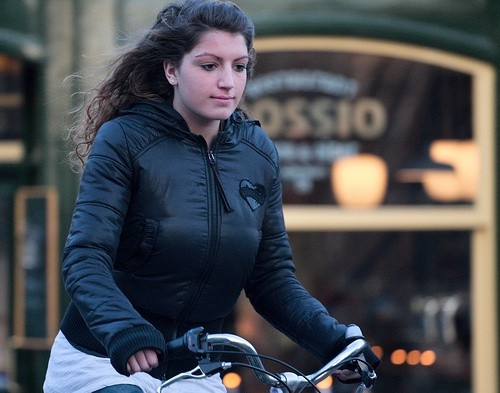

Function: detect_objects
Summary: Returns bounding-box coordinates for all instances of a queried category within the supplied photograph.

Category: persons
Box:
[42,0,370,393]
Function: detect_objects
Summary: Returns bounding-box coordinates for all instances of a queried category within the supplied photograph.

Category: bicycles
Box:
[155,323,378,393]
